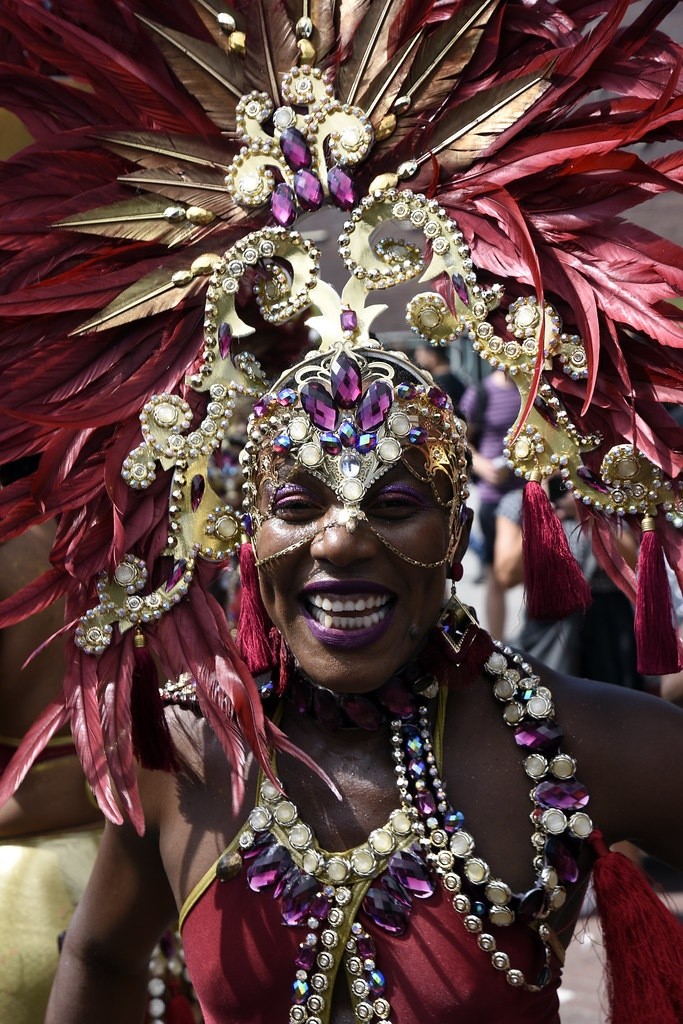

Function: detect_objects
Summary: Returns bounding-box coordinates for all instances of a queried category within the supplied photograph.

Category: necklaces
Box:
[255,626,458,731]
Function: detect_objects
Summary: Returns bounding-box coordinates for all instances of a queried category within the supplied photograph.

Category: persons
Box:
[416,339,680,700]
[42,346,681,1023]
